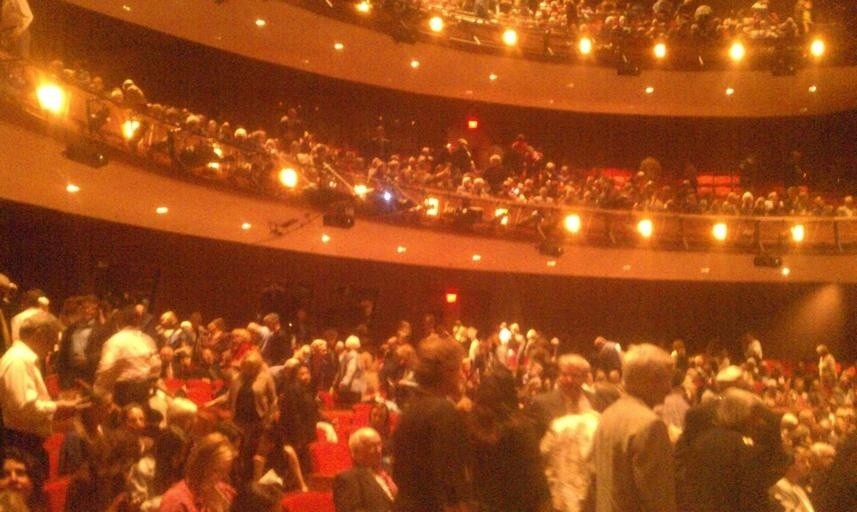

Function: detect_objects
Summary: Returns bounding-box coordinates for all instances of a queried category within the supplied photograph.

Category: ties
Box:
[376,470,398,496]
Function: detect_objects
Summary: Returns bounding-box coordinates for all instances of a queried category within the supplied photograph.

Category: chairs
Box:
[42,371,401,512]
[585,168,743,200]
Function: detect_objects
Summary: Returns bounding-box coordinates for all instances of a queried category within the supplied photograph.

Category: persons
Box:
[1,0,856,512]
